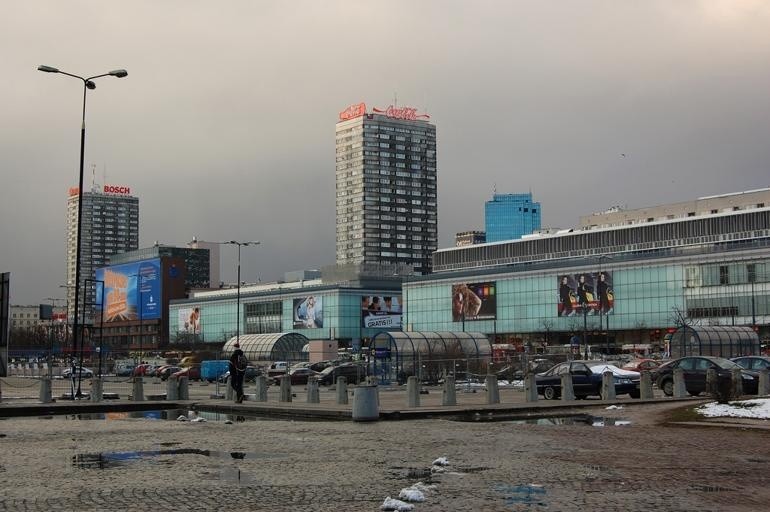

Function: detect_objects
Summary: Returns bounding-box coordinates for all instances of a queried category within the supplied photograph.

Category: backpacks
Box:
[234,354,247,374]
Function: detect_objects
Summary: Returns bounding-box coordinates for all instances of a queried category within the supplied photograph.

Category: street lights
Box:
[224,241,260,345]
[129,274,154,366]
[38,65,128,387]
[43,284,78,367]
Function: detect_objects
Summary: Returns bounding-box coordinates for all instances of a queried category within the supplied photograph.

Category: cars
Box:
[63,366,93,377]
[495,356,770,399]
[112,360,230,383]
[246,361,365,385]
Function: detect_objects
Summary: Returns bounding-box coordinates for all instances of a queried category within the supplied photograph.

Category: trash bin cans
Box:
[351,384,380,422]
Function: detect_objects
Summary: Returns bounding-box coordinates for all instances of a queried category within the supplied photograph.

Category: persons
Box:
[229,344,248,403]
[304,297,316,328]
[190,308,199,330]
[362,296,392,311]
[559,274,610,315]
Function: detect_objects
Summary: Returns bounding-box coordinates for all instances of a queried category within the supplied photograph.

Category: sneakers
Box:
[235,395,244,403]
